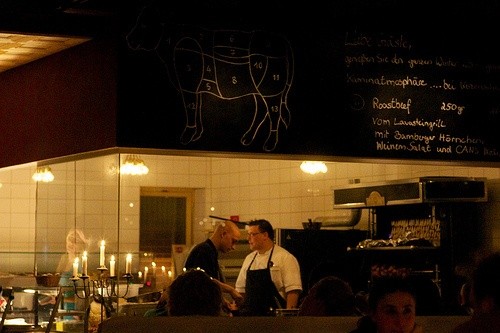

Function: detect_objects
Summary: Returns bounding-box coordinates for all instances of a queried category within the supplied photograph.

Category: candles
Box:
[109,254,116,276]
[70,257,78,277]
[125,254,132,273]
[82,250,89,275]
[99,239,106,267]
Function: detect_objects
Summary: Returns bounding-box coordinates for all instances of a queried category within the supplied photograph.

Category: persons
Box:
[144,267,244,316]
[167,220,243,317]
[448,252,500,333]
[233,219,303,317]
[350,276,423,333]
[56,229,91,320]
[297,276,360,318]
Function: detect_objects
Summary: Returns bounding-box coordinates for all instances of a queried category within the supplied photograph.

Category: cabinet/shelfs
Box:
[0,283,89,333]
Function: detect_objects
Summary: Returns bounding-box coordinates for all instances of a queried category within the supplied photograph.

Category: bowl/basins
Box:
[302,222,322,230]
[35,275,61,286]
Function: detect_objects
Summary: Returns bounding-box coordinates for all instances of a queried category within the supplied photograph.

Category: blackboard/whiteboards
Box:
[109,0,500,167]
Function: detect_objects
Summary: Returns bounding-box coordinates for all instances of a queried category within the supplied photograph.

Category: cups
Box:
[56,319,63,332]
[275,308,299,317]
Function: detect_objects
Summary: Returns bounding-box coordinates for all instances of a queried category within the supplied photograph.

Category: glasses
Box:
[247,231,262,238]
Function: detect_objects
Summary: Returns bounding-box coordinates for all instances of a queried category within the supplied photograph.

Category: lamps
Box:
[298,160,327,175]
[32,164,57,183]
[118,155,148,177]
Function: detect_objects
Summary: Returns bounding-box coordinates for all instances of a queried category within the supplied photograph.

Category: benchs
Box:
[96,314,473,333]
